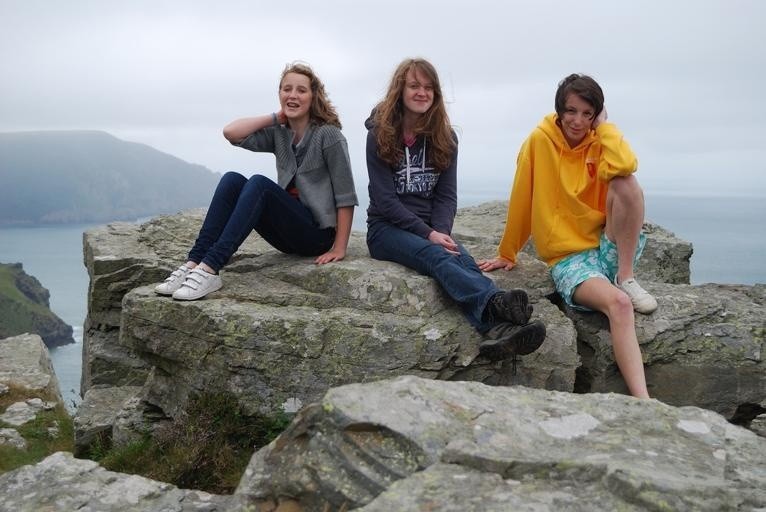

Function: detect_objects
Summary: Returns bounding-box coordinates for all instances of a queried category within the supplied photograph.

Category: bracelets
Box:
[271,111,279,127]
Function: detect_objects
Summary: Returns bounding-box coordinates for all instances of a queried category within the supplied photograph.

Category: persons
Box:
[152,60,360,303]
[470,70,660,401]
[362,55,548,361]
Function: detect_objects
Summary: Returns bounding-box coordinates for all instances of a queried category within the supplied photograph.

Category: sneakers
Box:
[153,263,193,295]
[493,289,534,326]
[172,265,224,300]
[614,273,658,314]
[479,320,546,362]
